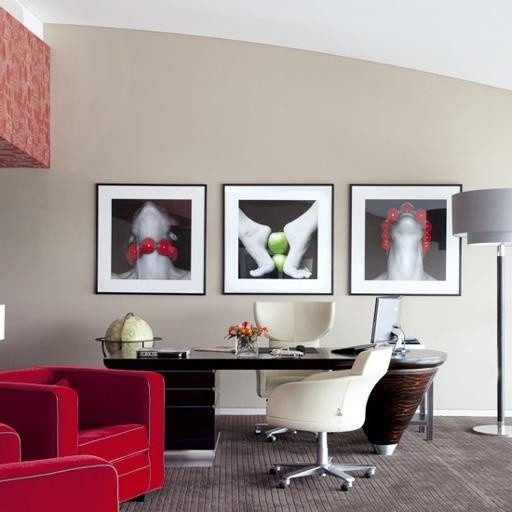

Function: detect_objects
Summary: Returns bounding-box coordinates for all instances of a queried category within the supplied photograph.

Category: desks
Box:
[103,346,448,466]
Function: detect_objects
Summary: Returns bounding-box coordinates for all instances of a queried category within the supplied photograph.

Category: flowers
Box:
[225,321,269,351]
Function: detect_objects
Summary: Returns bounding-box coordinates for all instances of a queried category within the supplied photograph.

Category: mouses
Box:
[296,345,305,353]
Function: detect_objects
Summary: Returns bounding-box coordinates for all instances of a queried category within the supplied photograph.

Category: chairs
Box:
[254,301,335,442]
[266,347,392,491]
[0,423,119,512]
[0,367,165,503]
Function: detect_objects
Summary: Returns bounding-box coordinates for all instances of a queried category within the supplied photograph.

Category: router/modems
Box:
[135,346,191,358]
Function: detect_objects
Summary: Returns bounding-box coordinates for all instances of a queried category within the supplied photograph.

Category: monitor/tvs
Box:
[370,296,405,352]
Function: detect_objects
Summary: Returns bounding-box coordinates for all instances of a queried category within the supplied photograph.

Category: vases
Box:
[235,336,258,357]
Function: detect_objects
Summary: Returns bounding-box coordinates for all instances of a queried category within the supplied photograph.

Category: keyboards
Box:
[331,344,377,356]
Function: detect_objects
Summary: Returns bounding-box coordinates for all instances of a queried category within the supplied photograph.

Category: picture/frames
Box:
[94,183,207,295]
[349,184,462,296]
[222,184,334,295]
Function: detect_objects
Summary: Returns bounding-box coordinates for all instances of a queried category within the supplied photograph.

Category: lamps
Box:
[453,187,512,439]
